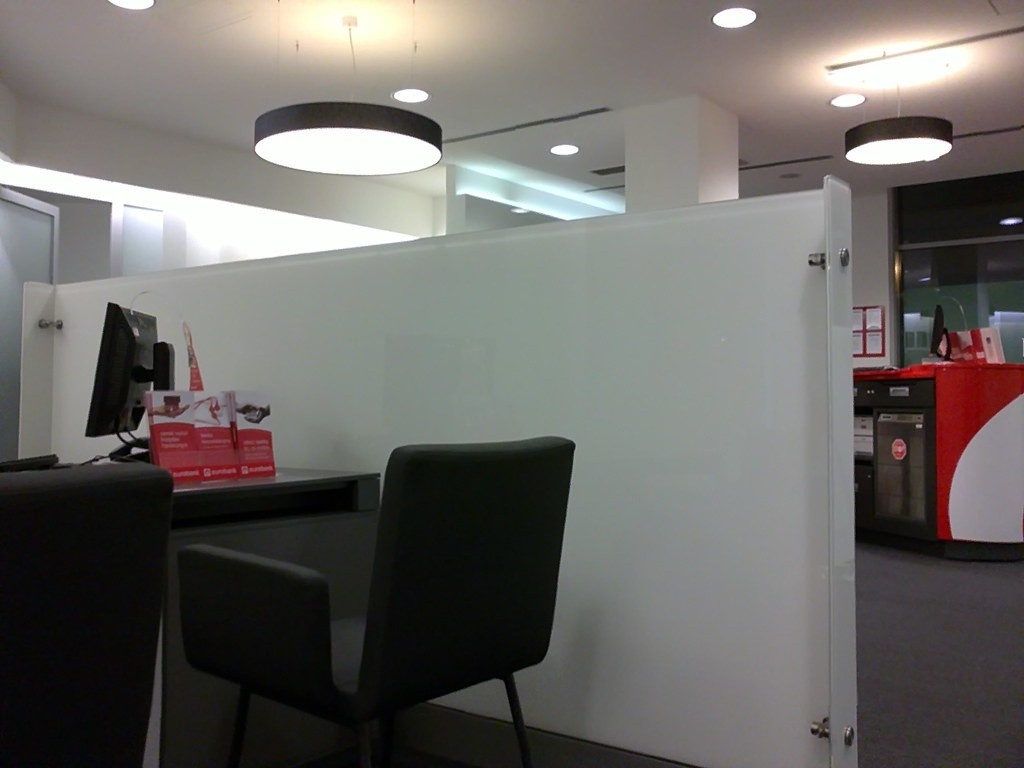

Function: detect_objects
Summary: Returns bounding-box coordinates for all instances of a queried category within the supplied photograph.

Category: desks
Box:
[0,466,381,710]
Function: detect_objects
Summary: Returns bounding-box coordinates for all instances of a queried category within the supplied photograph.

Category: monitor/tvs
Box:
[931,305,952,362]
[85,302,175,438]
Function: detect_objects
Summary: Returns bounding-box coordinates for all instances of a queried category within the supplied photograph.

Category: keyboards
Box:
[0,454,58,472]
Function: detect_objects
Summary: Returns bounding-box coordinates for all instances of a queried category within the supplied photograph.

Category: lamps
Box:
[254,0,443,176]
[844,71,953,165]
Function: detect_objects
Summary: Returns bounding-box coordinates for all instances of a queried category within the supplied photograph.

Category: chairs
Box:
[0,462,174,768]
[178,435,576,768]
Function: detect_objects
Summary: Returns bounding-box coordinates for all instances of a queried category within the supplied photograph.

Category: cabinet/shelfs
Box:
[853,361,1024,562]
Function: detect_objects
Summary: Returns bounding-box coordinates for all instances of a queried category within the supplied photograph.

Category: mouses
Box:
[883,366,897,371]
